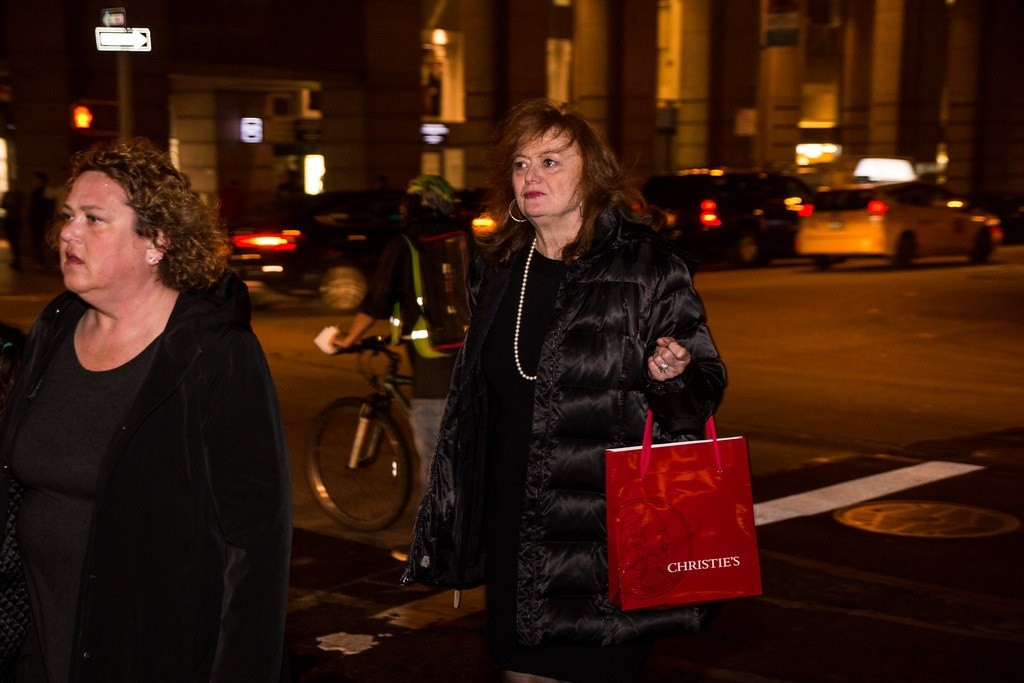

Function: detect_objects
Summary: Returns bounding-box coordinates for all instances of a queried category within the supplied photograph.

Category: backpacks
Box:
[401,227,483,359]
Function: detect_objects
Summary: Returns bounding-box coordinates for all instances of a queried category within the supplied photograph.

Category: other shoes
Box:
[391,545,410,562]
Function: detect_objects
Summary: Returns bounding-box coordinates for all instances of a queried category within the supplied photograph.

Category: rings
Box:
[659,363,669,374]
[677,348,688,361]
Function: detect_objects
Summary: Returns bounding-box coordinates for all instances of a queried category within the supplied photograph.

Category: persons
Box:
[0,171,59,270]
[0,138,293,683]
[331,175,482,568]
[400,98,729,683]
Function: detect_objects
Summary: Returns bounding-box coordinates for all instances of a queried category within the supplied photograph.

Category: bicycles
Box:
[303,332,416,532]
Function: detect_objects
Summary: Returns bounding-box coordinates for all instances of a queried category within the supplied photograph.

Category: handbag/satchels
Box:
[605,407,764,611]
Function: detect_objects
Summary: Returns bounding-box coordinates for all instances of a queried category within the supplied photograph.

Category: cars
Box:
[225,190,477,313]
[639,167,847,270]
[795,181,1004,270]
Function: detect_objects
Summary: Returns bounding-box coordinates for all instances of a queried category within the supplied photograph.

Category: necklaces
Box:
[513,236,538,381]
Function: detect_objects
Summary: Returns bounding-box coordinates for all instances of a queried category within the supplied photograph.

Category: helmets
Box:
[406,175,461,219]
